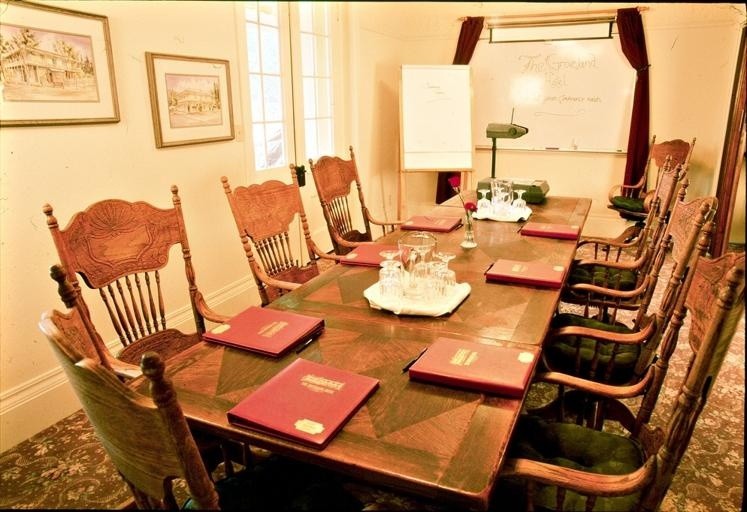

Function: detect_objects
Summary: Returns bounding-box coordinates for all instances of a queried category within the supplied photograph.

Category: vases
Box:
[460,214,477,249]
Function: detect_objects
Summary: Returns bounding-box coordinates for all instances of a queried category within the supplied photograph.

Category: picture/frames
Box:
[0,0,121,127]
[145,52,236,148]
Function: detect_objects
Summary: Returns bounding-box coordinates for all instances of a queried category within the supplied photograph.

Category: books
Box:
[225,358,379,451]
[399,214,462,233]
[408,336,541,400]
[202,306,324,358]
[521,221,579,241]
[486,258,565,289]
[341,245,413,269]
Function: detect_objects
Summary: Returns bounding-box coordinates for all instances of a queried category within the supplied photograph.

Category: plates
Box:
[471,204,533,222]
[363,277,472,317]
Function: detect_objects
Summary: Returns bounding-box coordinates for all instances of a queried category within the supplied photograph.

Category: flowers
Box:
[448,175,478,229]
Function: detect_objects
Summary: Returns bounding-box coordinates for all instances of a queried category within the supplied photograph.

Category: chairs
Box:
[501,235,746,511]
[606,135,696,221]
[43,186,233,473]
[539,188,719,383]
[556,153,681,324]
[220,164,346,306]
[38,265,365,512]
[309,146,406,266]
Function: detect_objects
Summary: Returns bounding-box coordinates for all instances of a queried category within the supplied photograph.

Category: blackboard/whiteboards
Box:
[467,38,637,155]
[398,63,474,172]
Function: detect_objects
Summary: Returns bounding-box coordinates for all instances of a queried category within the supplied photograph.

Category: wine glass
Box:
[476,178,527,216]
[379,231,456,304]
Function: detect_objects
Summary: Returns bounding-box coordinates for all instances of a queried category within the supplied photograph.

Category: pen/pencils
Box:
[296,331,323,354]
[455,223,463,230]
[403,346,427,372]
[516,225,523,233]
[545,147,561,150]
[484,262,495,275]
